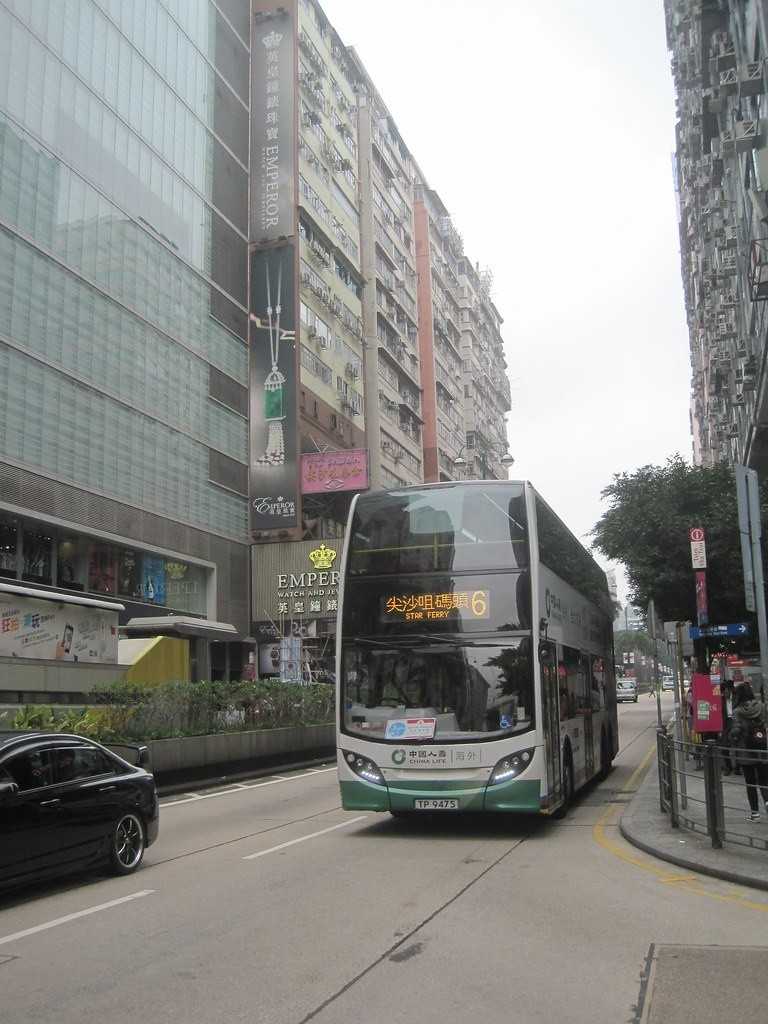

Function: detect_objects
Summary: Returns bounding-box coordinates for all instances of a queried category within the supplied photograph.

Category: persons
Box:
[558,687,577,722]
[648,683,656,698]
[380,663,420,708]
[686,680,743,776]
[591,679,601,713]
[729,682,768,824]
[145,574,155,605]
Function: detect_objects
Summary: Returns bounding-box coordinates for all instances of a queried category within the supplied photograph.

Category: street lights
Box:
[453,441,516,480]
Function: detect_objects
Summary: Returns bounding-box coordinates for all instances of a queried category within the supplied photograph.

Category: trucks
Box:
[662,675,675,691]
[616,677,639,702]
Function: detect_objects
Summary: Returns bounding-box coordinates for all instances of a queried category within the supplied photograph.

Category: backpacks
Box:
[746,717,768,758]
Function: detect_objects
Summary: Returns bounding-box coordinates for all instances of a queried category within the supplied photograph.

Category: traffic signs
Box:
[688,622,750,638]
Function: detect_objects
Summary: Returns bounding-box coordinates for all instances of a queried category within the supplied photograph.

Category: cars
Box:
[0,731,162,897]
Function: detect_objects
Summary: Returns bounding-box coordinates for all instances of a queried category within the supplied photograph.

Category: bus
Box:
[331,478,621,820]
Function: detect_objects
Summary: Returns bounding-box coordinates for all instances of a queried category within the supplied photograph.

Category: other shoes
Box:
[734,766,743,775]
[724,767,733,776]
[746,813,761,822]
[764,802,768,815]
